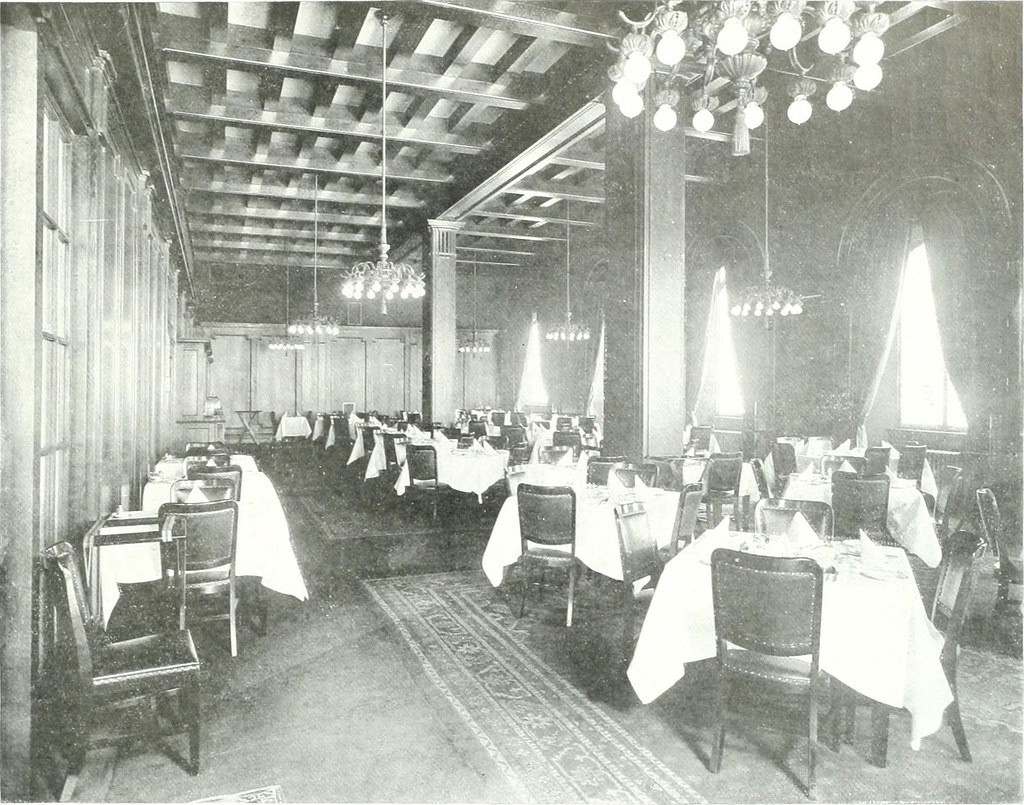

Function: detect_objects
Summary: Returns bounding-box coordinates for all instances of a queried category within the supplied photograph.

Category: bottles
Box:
[116,504,124,515]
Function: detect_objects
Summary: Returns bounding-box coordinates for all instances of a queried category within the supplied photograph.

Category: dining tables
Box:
[233,410,263,448]
[274,416,312,443]
[313,410,945,769]
[90,451,314,642]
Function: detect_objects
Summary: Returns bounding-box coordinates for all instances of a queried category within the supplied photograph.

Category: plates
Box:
[111,512,132,519]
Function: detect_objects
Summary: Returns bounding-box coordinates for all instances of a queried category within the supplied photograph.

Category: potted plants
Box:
[267,255,306,356]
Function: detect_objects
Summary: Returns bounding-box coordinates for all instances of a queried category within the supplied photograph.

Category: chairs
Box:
[181,440,229,453]
[932,464,964,534]
[307,410,314,426]
[706,544,828,799]
[976,486,1023,649]
[37,497,245,776]
[270,411,280,441]
[830,529,990,768]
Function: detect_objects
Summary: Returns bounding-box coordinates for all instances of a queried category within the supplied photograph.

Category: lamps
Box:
[607,0,894,320]
[458,253,492,353]
[546,196,592,343]
[287,174,343,338]
[340,9,428,317]
